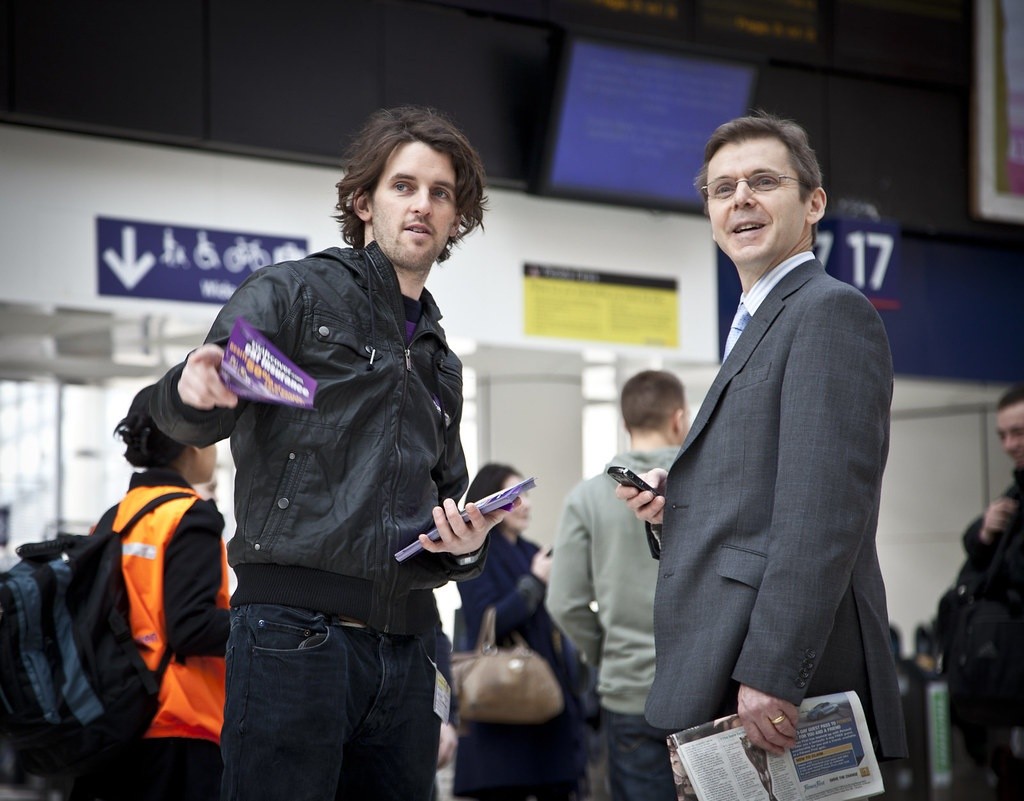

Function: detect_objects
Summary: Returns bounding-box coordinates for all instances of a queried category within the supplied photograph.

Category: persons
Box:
[86,384,229,801]
[615,117,912,801]
[454,464,593,801]
[963,382,1024,800]
[545,370,691,801]
[149,107,522,801]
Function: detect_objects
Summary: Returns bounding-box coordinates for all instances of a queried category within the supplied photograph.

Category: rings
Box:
[771,713,785,724]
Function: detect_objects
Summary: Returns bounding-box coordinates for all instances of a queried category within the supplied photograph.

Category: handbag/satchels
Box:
[449,608,564,722]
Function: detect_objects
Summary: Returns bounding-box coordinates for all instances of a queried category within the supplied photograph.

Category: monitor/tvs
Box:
[532,28,768,215]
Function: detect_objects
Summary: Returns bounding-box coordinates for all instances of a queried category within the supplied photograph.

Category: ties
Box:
[723,300,750,361]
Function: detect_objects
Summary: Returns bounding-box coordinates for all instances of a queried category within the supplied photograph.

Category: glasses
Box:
[700,170,814,199]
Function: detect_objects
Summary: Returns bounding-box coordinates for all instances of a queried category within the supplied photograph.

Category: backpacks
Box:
[0,492,202,794]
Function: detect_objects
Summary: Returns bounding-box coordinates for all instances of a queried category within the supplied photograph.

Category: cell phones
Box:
[608,466,660,501]
[545,547,554,557]
